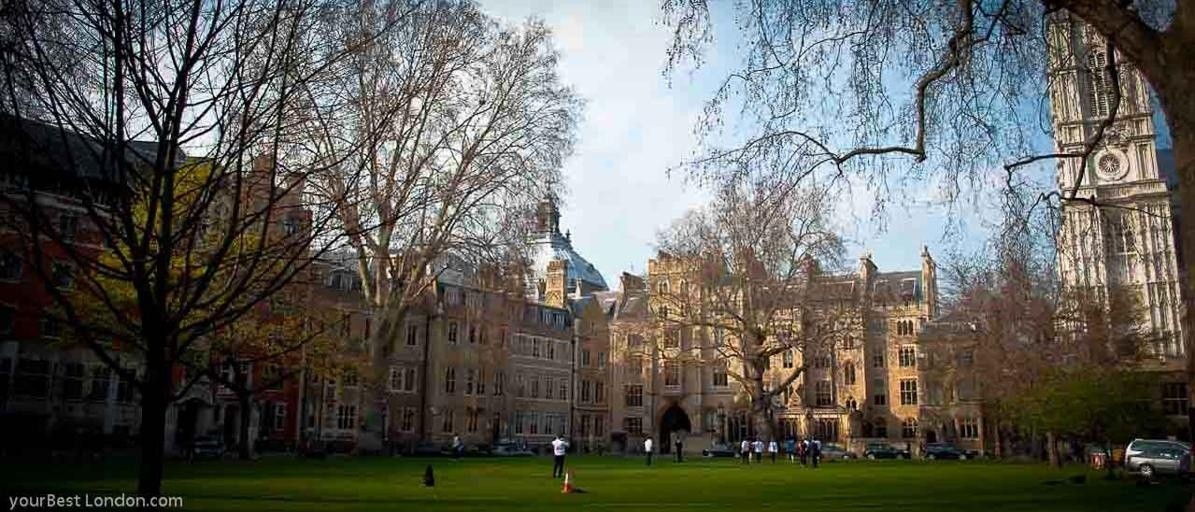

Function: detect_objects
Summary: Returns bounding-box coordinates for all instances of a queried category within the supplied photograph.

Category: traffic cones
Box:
[559,469,574,494]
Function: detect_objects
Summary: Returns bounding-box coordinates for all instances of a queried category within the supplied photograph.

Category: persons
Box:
[739,435,825,468]
[550,431,571,478]
[644,434,655,467]
[451,430,464,459]
[674,434,683,463]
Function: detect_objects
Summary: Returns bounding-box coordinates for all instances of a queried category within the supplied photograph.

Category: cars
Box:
[924,443,979,462]
[702,442,744,458]
[400,438,554,458]
[816,444,857,462]
[1123,437,1190,478]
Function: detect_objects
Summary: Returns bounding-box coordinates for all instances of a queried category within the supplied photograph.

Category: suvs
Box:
[863,441,910,461]
